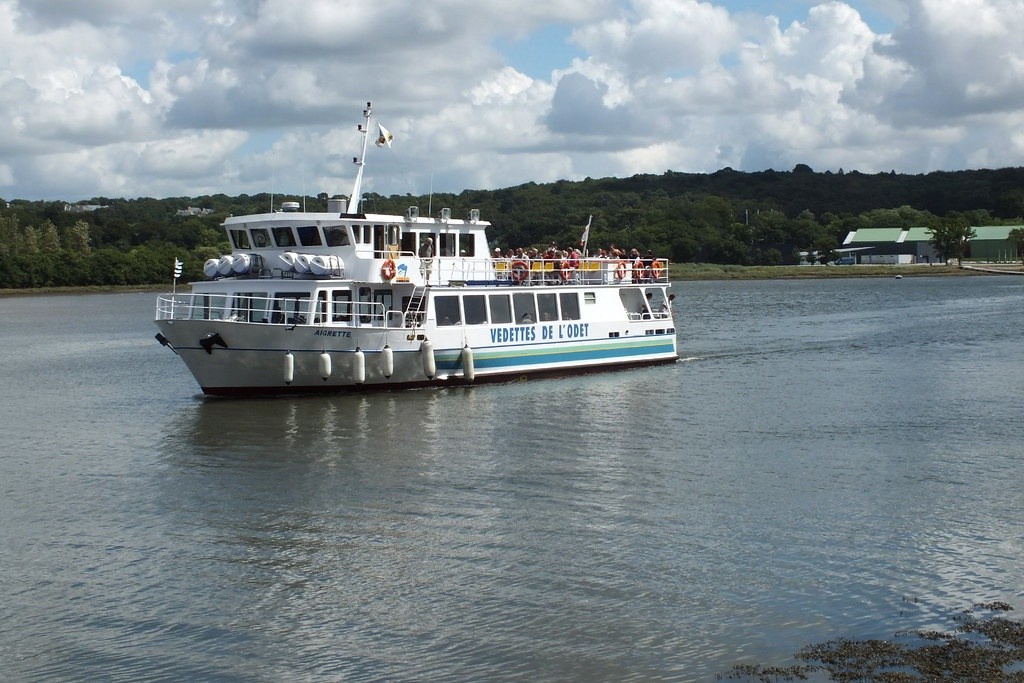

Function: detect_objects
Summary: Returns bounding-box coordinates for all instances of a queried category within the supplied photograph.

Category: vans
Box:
[834,257,855,266]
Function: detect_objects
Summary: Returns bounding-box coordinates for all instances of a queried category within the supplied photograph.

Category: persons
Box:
[492,241,654,286]
[271,300,281,323]
[420,237,435,287]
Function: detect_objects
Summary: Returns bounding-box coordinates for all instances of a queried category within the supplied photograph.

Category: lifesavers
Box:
[560,262,571,278]
[634,262,645,278]
[651,262,661,277]
[512,261,529,279]
[381,260,397,279]
[617,262,627,278]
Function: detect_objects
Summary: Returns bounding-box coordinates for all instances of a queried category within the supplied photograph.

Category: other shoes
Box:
[426,285,432,287]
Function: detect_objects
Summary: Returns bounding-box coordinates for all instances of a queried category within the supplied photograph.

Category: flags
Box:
[375,122,394,152]
[580,214,593,245]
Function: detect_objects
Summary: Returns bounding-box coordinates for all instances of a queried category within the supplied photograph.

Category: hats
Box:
[494,248,501,252]
[551,242,557,246]
[567,247,573,252]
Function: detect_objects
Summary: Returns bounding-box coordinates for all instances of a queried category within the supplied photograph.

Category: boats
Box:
[153,99,681,399]
